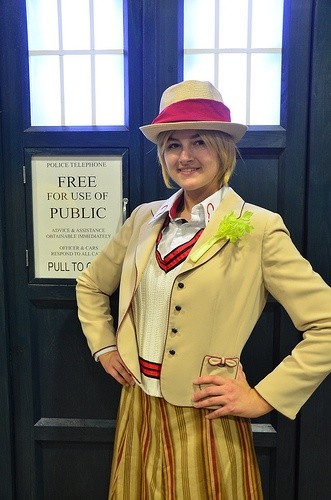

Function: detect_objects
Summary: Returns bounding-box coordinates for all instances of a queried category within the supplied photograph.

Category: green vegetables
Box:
[190,211,255,263]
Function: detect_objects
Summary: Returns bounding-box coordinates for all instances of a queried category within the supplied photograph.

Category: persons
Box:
[76,81,331,500]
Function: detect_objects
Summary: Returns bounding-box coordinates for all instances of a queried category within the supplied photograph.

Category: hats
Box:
[139,79,249,144]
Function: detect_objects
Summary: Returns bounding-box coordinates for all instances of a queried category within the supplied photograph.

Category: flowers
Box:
[214,210,255,247]
